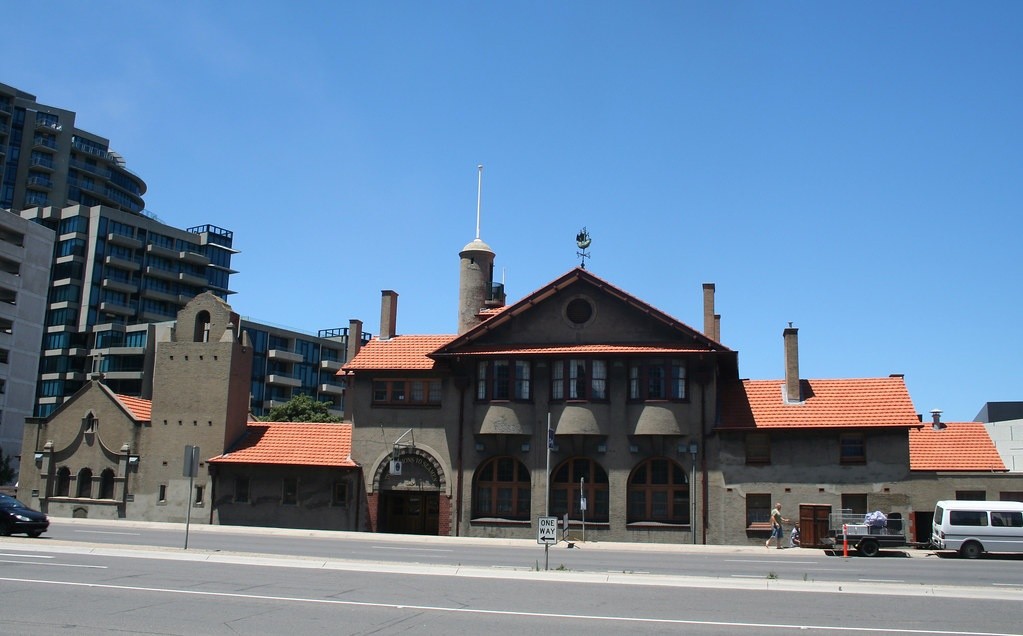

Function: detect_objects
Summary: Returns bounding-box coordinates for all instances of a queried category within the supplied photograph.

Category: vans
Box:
[930,501,1023,559]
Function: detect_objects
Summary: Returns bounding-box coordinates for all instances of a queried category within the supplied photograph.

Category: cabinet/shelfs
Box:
[799,503,833,549]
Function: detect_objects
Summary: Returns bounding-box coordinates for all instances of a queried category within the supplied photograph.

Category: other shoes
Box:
[765,540,769,548]
[777,546,783,549]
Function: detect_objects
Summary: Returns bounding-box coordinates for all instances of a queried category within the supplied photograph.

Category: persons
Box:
[791,522,801,548]
[765,503,789,549]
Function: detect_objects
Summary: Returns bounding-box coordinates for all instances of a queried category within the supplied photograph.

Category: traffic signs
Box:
[538,516,562,548]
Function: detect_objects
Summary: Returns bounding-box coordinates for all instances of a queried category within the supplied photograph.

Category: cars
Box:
[0,493,49,538]
